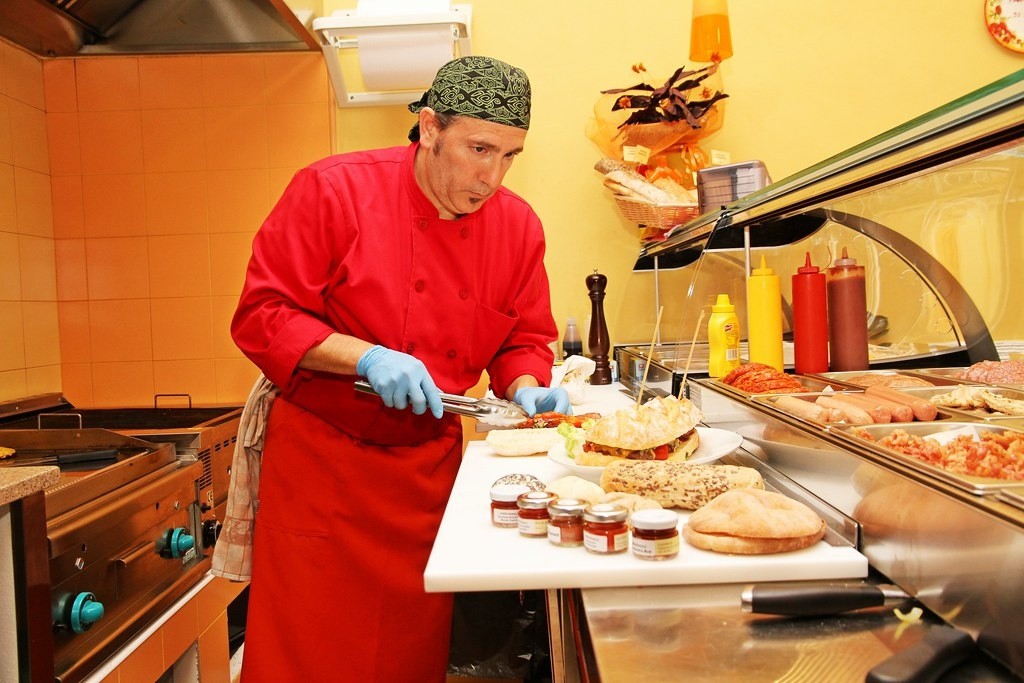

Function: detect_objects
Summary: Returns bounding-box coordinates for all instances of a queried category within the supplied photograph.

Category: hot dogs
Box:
[486,412,601,455]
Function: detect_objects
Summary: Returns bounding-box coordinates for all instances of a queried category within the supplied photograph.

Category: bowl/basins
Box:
[707,367,1024,513]
[627,340,795,375]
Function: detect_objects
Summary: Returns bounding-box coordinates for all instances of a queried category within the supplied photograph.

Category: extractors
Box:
[0,0,323,60]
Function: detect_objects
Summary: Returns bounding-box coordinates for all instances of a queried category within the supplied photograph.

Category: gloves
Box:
[514,385,574,419]
[355,345,444,418]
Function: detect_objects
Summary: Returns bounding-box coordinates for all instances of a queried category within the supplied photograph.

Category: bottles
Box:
[708,294,741,377]
[826,245,869,372]
[562,319,582,361]
[747,255,784,376]
[792,251,830,376]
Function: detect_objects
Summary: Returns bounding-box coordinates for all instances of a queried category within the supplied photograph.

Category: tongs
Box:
[354,379,530,426]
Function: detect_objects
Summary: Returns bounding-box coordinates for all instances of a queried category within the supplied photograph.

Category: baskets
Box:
[613,193,702,230]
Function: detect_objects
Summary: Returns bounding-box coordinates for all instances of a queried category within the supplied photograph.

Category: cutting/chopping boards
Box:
[424,441,869,593]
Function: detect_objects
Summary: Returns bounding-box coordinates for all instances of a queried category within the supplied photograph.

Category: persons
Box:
[229,54,575,683]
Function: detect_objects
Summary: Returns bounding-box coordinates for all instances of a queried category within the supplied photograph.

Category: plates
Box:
[550,426,743,485]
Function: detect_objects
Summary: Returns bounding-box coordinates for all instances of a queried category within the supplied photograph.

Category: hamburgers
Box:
[571,394,702,467]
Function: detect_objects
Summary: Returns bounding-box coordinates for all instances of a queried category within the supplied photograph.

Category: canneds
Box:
[489,485,680,562]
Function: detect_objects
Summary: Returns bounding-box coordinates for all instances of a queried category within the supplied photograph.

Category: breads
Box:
[594,156,697,204]
[681,486,826,555]
[600,458,764,511]
[491,474,663,529]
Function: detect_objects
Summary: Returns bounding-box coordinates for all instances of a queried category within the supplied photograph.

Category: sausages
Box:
[773,385,938,426]
